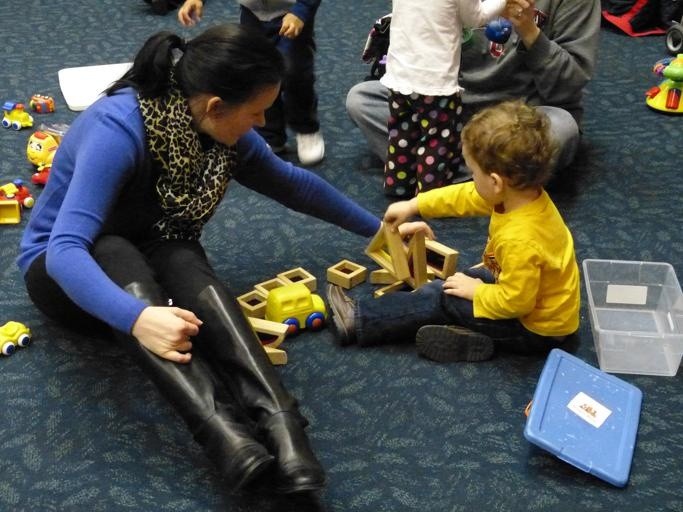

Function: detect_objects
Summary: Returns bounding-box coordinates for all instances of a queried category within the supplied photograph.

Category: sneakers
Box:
[297,130,324,164]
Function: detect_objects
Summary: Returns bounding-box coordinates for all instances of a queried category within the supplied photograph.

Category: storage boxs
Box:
[582,258,683,377]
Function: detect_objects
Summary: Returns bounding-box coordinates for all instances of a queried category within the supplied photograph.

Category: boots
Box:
[191,283,325,494]
[108,280,274,495]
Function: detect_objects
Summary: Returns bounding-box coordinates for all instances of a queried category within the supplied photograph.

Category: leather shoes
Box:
[415,324,494,363]
[327,282,357,346]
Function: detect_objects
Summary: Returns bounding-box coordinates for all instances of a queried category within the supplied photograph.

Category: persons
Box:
[176,0,328,164]
[324,93,584,364]
[345,2,603,194]
[15,19,436,505]
[378,1,508,202]
